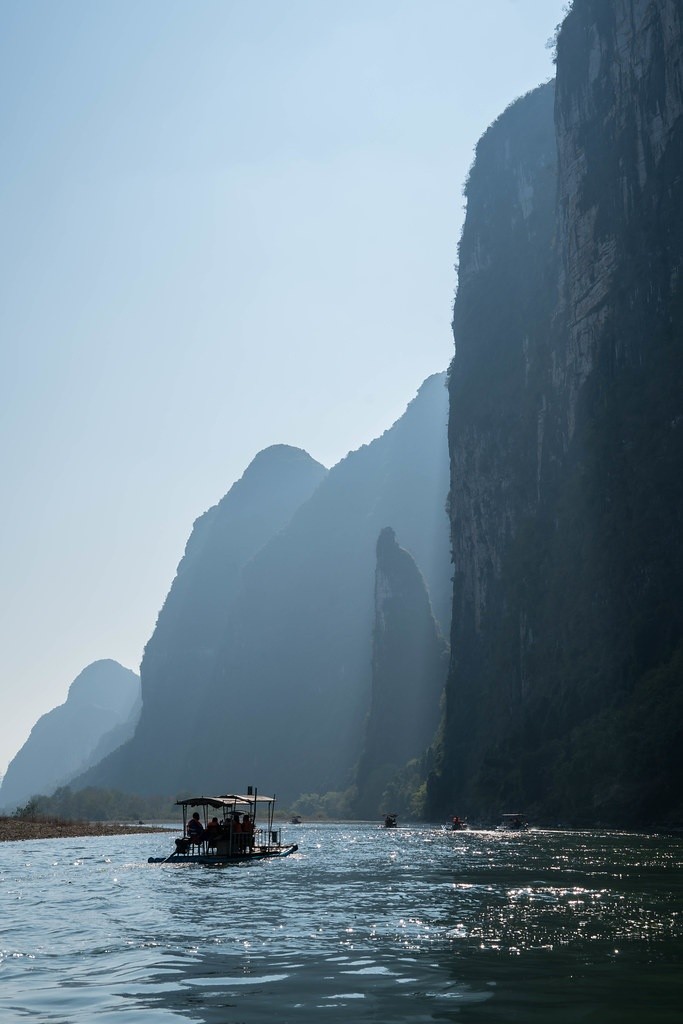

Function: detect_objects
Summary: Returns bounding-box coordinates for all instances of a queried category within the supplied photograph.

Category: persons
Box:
[453,816,464,830]
[384,816,397,828]
[499,818,523,829]
[187,812,255,854]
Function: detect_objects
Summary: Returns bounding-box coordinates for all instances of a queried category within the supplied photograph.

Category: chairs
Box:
[186,826,214,855]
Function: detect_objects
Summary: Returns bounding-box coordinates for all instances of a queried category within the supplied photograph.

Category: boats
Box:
[286,815,302,824]
[382,813,399,827]
[148,785,298,863]
[495,814,528,832]
[446,815,468,830]
